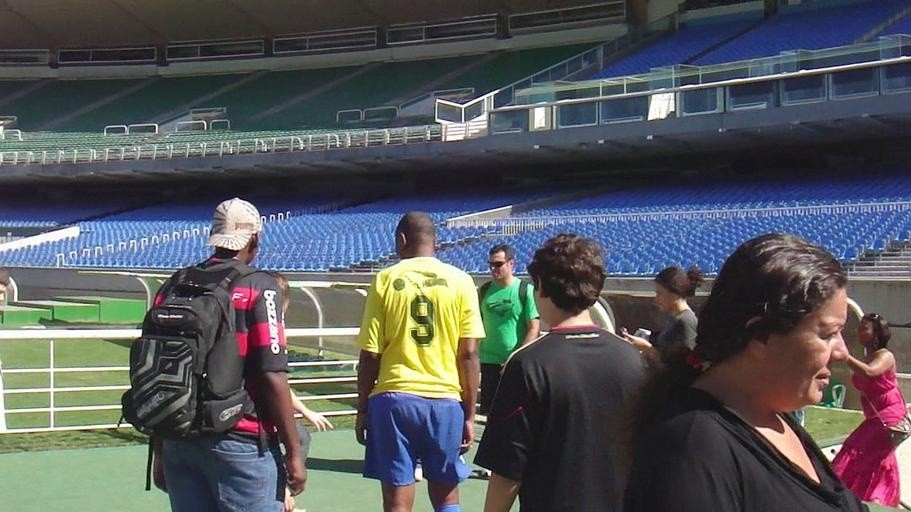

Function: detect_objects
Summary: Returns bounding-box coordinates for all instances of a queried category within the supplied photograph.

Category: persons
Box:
[476,244,541,478]
[355,212,488,512]
[622,265,704,378]
[154,198,307,511]
[258,269,334,511]
[474,234,653,512]
[629,234,873,512]
[832,296,907,509]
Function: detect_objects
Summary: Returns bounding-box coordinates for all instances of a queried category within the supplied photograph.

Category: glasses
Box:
[487,261,508,267]
[869,314,880,323]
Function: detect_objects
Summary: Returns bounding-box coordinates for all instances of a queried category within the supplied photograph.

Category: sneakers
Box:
[481,468,493,477]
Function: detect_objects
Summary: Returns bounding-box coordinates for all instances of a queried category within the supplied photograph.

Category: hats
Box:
[206,197,262,251]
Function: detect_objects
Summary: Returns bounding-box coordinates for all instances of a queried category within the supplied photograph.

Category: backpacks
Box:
[122,262,250,437]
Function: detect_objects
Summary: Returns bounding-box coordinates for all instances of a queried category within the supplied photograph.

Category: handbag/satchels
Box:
[886,412,911,443]
